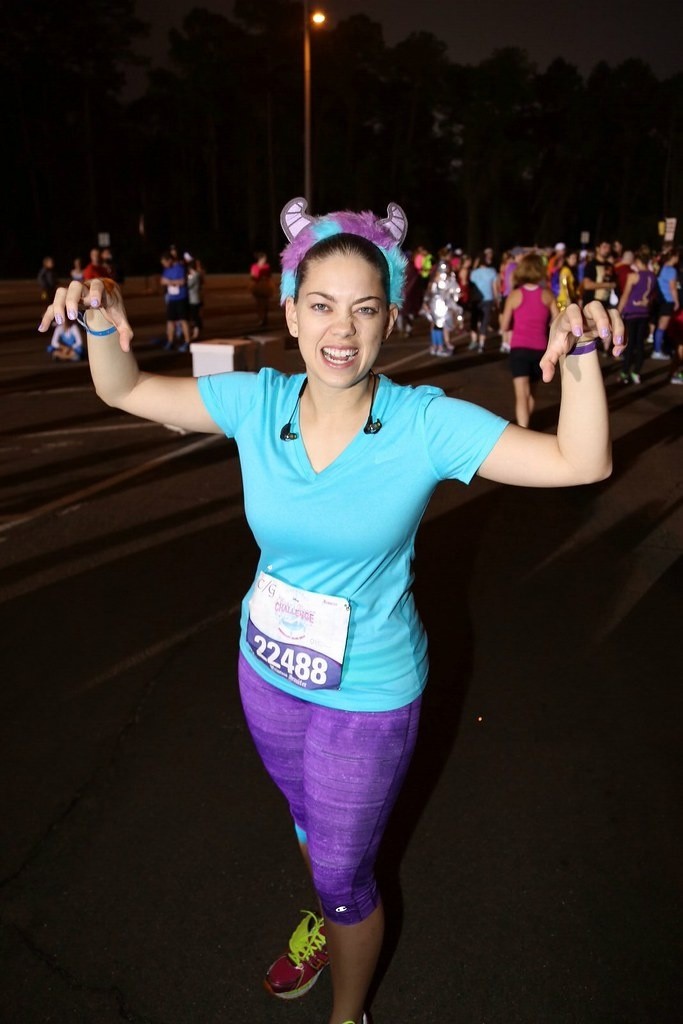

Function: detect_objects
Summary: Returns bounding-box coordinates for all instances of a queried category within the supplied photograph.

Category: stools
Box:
[190,334,287,377]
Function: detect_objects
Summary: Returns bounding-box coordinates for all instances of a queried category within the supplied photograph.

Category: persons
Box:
[248,249,274,329]
[498,253,562,431]
[36,212,626,1023]
[39,242,205,352]
[402,238,683,387]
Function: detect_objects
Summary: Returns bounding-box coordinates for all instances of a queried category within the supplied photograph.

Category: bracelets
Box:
[566,341,597,356]
[73,310,118,337]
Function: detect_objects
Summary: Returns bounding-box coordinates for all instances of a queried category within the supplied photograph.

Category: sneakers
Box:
[264,910,330,1000]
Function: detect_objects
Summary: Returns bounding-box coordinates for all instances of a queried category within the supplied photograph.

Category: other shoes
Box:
[670,373,683,385]
[616,370,641,384]
[651,351,670,360]
[500,345,511,353]
[478,347,483,353]
[180,343,188,352]
[165,342,173,351]
[468,341,477,349]
[430,349,452,356]
[647,337,655,343]
[447,344,455,351]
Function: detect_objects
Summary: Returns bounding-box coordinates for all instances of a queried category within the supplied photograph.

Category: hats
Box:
[455,248,462,255]
[484,248,493,253]
[579,249,588,261]
[555,243,566,251]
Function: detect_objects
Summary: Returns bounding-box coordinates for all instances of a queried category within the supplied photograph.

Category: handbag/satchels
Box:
[464,270,484,310]
[610,283,618,305]
